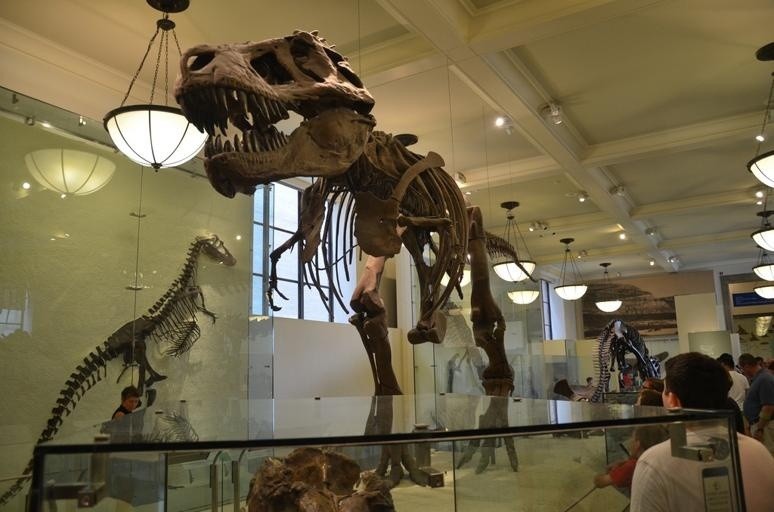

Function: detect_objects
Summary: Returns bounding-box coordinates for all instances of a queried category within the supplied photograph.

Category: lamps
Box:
[507,282,540,305]
[609,184,625,197]
[103,0,210,171]
[545,100,562,126]
[554,238,588,301]
[493,201,536,282]
[750,225,773,253]
[594,262,623,313]
[578,192,586,203]
[647,226,657,237]
[529,222,550,232]
[746,42,774,191]
[751,209,773,282]
[753,245,774,300]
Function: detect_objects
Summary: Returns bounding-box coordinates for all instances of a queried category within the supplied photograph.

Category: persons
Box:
[586,352,774,512]
[112,386,139,420]
